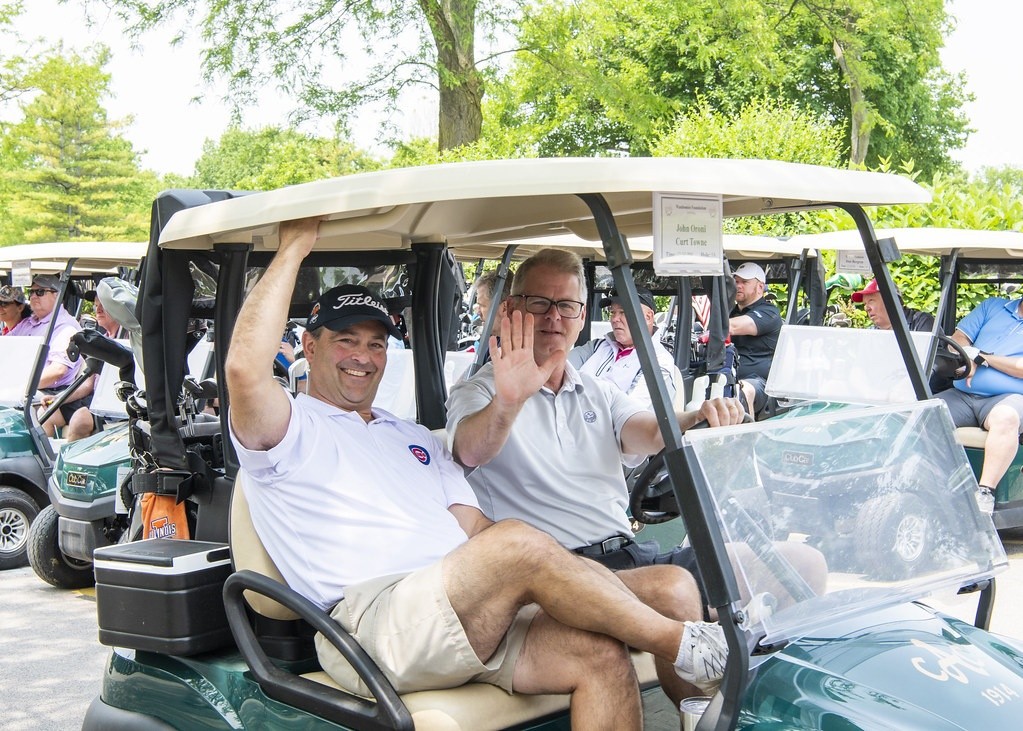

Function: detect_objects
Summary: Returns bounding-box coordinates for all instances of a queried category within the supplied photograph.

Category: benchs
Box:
[215,458,715,731]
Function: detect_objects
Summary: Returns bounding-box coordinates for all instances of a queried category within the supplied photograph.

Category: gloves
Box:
[959,346,994,368]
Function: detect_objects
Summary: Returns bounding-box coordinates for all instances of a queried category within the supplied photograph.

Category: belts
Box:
[573,537,631,556]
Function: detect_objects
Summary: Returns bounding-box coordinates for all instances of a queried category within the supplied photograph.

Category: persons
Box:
[851,278,946,352]
[0,284,33,338]
[3,274,84,397]
[225,214,785,730]
[930,297,1023,516]
[38,287,134,444]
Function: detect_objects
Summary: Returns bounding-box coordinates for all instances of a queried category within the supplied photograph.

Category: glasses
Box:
[27,289,57,296]
[511,294,586,320]
[0,302,16,306]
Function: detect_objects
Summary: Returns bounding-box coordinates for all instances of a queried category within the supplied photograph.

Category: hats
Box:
[851,278,904,302]
[306,285,402,341]
[0,285,25,302]
[731,262,766,284]
[32,274,62,292]
[597,283,656,311]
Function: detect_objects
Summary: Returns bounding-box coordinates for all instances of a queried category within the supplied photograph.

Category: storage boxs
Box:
[91,540,232,655]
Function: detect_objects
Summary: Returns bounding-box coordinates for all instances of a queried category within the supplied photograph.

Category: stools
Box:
[953,425,988,449]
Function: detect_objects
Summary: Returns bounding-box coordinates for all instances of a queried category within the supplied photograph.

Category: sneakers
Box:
[975,487,996,517]
[674,592,778,696]
